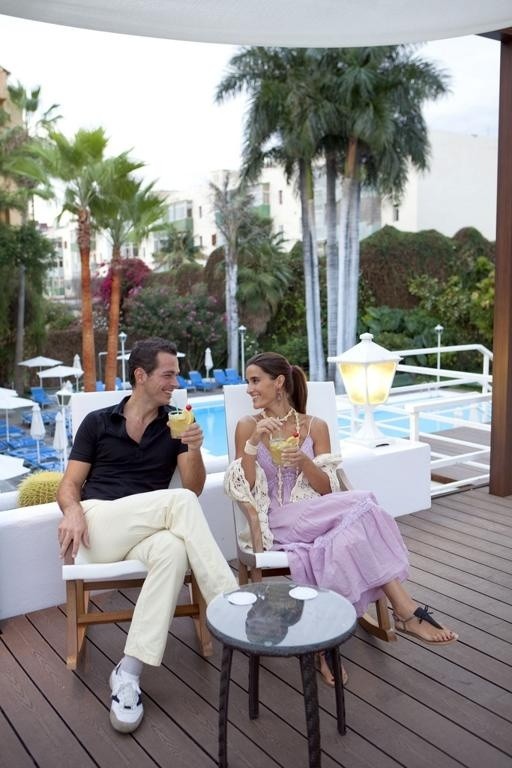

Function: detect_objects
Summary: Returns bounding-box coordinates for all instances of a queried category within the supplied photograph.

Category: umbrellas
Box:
[203,346,214,377]
[0,388,19,397]
[35,364,84,387]
[117,348,187,360]
[29,402,46,464]
[17,355,62,389]
[0,396,34,451]
[52,410,68,473]
[71,351,85,393]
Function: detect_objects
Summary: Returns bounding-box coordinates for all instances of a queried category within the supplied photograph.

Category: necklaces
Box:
[260,407,300,508]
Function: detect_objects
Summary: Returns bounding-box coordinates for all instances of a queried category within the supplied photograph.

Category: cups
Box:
[268,439,298,465]
[168,410,190,440]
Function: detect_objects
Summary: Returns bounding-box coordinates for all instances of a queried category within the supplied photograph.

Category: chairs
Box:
[60,385,216,669]
[222,382,396,645]
[2,368,250,482]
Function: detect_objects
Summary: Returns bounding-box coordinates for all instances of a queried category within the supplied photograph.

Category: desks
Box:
[206,581,358,768]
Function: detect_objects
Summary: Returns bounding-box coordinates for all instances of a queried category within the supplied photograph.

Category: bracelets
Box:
[244,440,259,456]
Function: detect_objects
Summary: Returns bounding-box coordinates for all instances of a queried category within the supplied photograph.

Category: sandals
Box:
[392,604,459,645]
[317,652,349,688]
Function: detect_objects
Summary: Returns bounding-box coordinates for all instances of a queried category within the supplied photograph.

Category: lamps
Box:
[325,332,404,447]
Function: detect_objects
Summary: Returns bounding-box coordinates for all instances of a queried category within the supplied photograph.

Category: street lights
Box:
[434,322,444,391]
[238,324,248,381]
[118,330,128,391]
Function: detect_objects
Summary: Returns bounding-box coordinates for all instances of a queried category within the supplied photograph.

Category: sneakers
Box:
[109,658,144,734]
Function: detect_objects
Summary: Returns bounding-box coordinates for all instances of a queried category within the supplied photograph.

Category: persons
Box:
[229,348,461,691]
[52,338,244,734]
